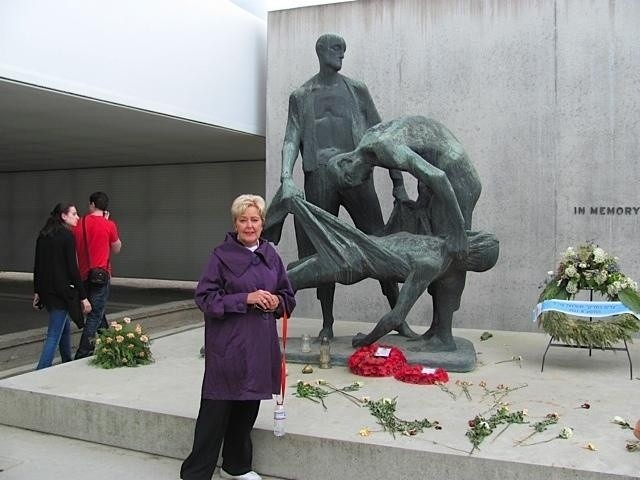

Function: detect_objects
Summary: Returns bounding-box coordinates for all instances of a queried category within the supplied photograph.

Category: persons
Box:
[324,115,481,352]
[180,194,296,479]
[280,33,420,344]
[31,192,121,369]
[261,186,499,347]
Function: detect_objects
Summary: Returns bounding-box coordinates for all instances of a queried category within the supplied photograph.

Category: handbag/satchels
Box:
[88,268,109,285]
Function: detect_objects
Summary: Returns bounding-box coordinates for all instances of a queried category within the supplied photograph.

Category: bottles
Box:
[320,337,331,370]
[274,398,289,439]
[301,334,312,354]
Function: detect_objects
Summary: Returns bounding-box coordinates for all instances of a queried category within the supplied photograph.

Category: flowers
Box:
[479,380,487,388]
[499,383,510,392]
[361,397,372,402]
[560,427,570,438]
[537,239,639,350]
[348,347,404,378]
[359,426,370,437]
[520,409,526,414]
[90,314,156,371]
[297,380,312,388]
[380,398,398,406]
[394,364,448,386]
[496,401,510,414]
[356,380,364,388]
[315,378,325,386]
[547,411,558,419]
[455,379,475,387]
[611,416,629,428]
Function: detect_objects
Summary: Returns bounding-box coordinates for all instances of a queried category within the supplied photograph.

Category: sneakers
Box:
[220,467,262,480]
